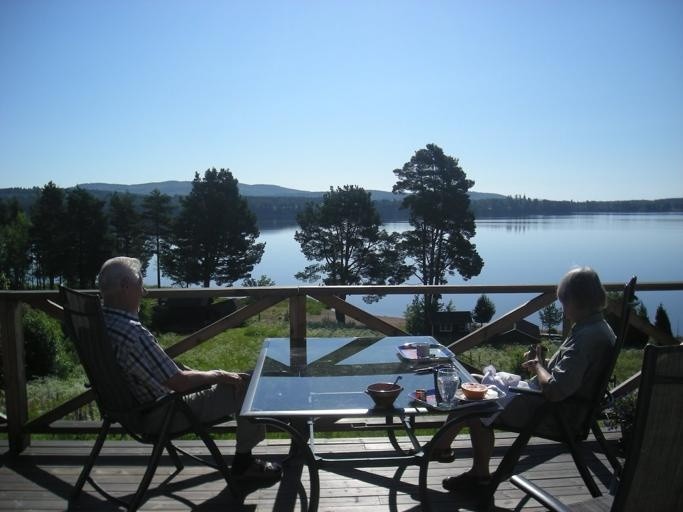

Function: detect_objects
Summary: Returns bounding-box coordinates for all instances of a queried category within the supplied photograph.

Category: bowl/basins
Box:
[461,383,488,399]
[365,383,403,405]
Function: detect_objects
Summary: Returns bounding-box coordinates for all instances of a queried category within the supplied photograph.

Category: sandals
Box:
[441,474,491,490]
[409,447,455,463]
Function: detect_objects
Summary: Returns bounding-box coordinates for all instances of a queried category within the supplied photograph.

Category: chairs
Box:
[476,274,683,511]
[58,283,239,511]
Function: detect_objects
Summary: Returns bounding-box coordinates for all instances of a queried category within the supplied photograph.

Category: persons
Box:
[96,257,284,485]
[405,267,617,490]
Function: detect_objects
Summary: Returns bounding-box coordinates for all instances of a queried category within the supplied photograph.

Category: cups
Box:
[416,389,426,402]
[432,365,455,408]
[417,342,430,359]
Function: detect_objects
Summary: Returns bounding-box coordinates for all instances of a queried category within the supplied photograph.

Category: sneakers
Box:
[231,458,284,484]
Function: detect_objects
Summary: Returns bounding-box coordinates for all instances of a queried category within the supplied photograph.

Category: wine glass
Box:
[436,367,460,408]
